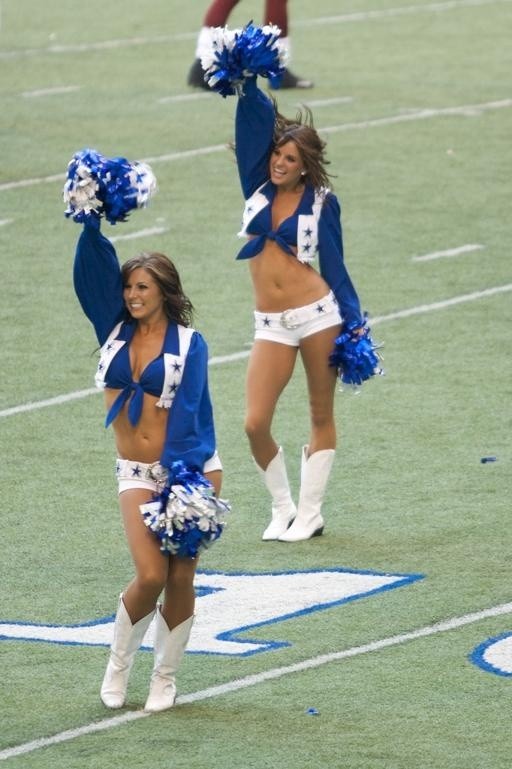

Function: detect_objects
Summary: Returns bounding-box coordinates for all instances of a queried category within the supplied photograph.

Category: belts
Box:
[117,459,168,486]
[254,293,336,327]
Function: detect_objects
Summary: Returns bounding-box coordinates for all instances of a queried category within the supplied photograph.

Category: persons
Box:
[191,21,386,541]
[62,149,231,713]
[186,0,312,93]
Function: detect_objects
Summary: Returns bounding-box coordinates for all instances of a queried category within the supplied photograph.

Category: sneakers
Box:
[186,59,210,90]
[281,70,314,89]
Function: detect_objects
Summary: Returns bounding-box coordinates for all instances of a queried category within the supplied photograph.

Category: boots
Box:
[251,445,296,542]
[143,604,196,714]
[99,593,157,710]
[279,444,336,543]
